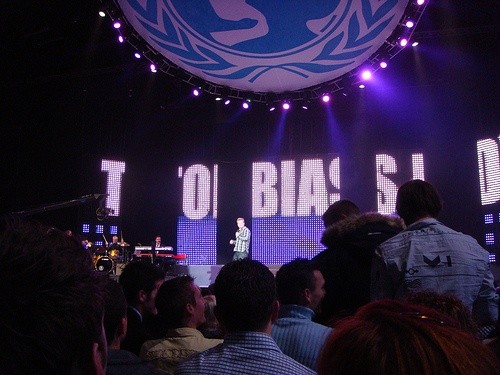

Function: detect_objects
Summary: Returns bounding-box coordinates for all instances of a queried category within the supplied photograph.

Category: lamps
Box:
[300,97,314,112]
[342,86,352,97]
[96,1,112,21]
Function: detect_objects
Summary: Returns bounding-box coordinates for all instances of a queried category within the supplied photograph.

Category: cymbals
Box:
[92,245,106,253]
[117,242,130,246]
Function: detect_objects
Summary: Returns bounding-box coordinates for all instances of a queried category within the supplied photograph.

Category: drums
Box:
[110,250,119,259]
[95,256,114,273]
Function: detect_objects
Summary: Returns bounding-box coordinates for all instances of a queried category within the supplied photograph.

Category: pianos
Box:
[135,246,173,251]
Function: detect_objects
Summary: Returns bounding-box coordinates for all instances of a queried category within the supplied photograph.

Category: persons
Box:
[312,199,403,326]
[0,221,108,375]
[106,235,122,257]
[119,261,165,356]
[315,298,500,375]
[140,275,224,375]
[201,295,222,338]
[174,260,318,375]
[229,218,251,261]
[270,257,334,371]
[373,179,500,336]
[85,240,93,249]
[98,274,154,374]
[153,235,165,254]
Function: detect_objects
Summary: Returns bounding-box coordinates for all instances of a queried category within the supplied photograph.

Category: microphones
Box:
[237,229,239,232]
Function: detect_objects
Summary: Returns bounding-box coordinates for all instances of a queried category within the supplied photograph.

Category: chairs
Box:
[172,253,188,277]
